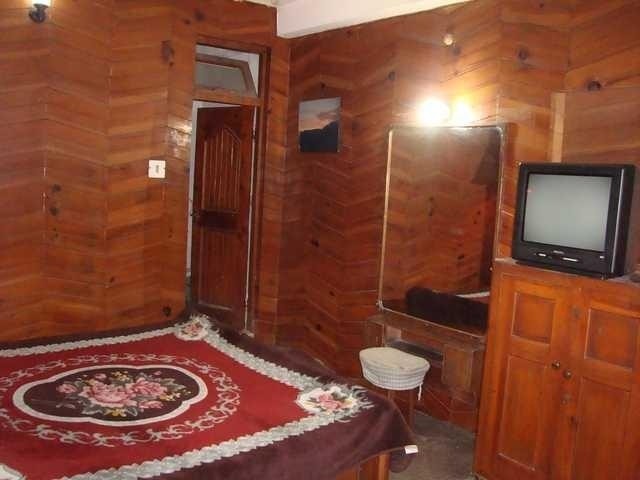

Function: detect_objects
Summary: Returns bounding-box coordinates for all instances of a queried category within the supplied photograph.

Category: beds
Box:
[0,316,419,479]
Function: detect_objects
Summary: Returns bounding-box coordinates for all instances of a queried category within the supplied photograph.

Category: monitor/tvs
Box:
[511,162,640,280]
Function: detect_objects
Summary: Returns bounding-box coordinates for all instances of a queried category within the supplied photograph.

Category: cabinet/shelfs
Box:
[363,258,640,480]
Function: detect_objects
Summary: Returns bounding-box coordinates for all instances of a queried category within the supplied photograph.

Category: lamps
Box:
[27,1,53,25]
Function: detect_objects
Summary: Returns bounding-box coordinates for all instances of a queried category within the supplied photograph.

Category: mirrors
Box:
[381,125,508,334]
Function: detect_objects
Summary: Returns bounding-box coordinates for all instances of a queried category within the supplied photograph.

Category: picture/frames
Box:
[298,98,339,153]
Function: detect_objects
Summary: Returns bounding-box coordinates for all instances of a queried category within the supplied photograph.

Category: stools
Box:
[357,342,429,436]
